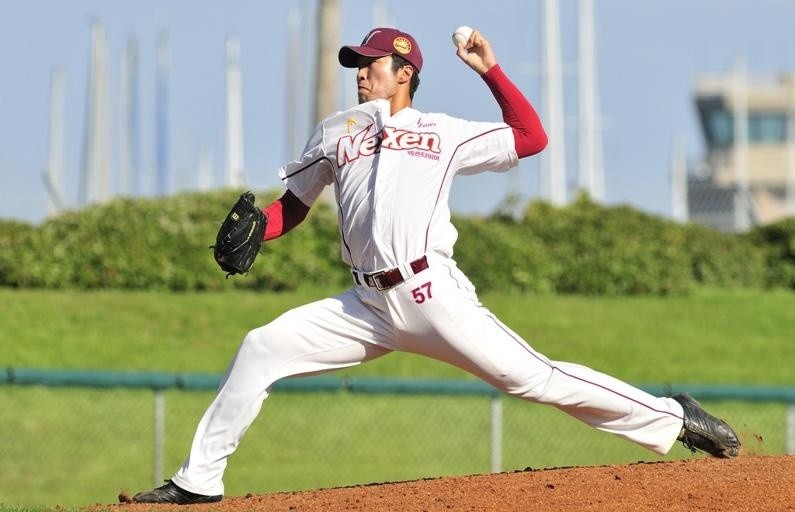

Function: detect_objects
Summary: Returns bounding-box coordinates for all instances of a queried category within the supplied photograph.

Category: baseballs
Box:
[452,26,475,48]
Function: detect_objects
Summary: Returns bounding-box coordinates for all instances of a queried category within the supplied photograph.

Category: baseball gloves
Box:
[212,191,267,274]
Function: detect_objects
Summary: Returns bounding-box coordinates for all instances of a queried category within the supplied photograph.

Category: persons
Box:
[132,28,743,503]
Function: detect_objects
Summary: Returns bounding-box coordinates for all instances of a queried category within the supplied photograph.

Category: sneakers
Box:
[672,392,740,457]
[133,480,225,503]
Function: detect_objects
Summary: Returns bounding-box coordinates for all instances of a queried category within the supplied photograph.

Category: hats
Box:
[338,27,423,72]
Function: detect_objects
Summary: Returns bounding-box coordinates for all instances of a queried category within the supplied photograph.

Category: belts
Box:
[353,255,429,292]
